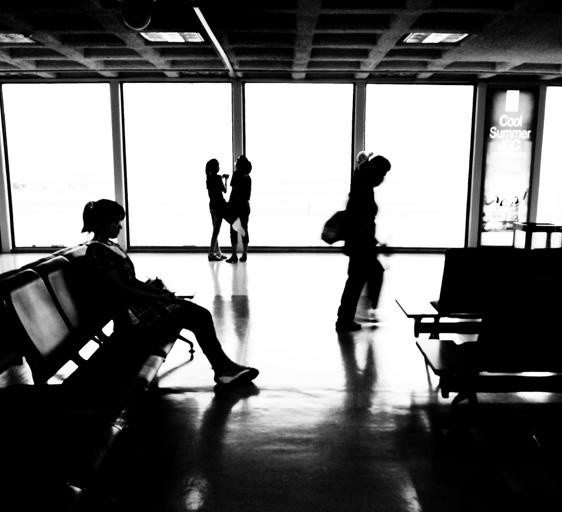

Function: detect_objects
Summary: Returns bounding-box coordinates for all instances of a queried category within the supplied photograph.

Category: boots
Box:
[226,231,238,263]
[239,231,249,262]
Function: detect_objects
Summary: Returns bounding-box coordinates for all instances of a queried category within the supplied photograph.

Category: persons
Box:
[206,159,229,261]
[335,155,391,332]
[225,155,252,262]
[81,200,258,387]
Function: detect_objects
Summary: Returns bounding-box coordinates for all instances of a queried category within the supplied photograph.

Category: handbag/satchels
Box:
[115,278,185,352]
[320,209,347,247]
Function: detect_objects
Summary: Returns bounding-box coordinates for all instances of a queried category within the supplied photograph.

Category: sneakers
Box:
[208,254,227,261]
[213,367,259,386]
[335,307,380,332]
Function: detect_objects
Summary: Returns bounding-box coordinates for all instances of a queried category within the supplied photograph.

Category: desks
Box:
[512,222,562,248]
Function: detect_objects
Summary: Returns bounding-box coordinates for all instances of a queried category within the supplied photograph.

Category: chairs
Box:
[0,242,194,409]
[393,249,562,440]
